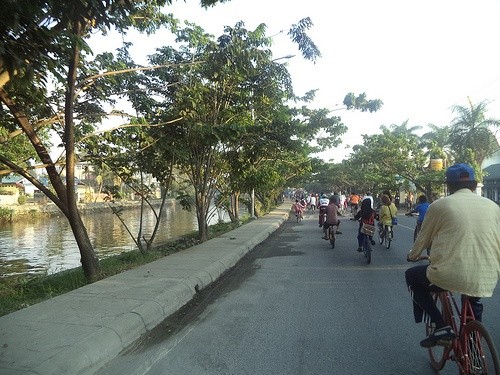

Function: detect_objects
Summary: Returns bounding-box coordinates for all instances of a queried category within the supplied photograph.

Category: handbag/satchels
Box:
[392,217,398,225]
[360,223,376,237]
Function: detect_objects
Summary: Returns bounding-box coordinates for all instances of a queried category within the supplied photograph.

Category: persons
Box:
[289,189,430,252]
[405,162,500,375]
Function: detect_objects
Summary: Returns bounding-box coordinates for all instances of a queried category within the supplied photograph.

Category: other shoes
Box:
[322,236,327,239]
[357,248,364,252]
[319,225,322,227]
[379,238,383,244]
[369,237,372,241]
[336,231,342,234]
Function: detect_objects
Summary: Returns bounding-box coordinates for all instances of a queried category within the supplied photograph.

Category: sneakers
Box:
[420,325,455,348]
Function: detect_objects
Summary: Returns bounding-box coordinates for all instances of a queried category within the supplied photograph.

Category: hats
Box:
[322,194,328,197]
[444,163,475,184]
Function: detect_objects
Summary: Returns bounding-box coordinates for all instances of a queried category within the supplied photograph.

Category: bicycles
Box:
[350,218,372,264]
[418,255,500,375]
[323,214,339,248]
[296,207,304,223]
[376,210,393,249]
[321,208,327,225]
[351,204,358,216]
[310,205,315,215]
[406,212,432,256]
